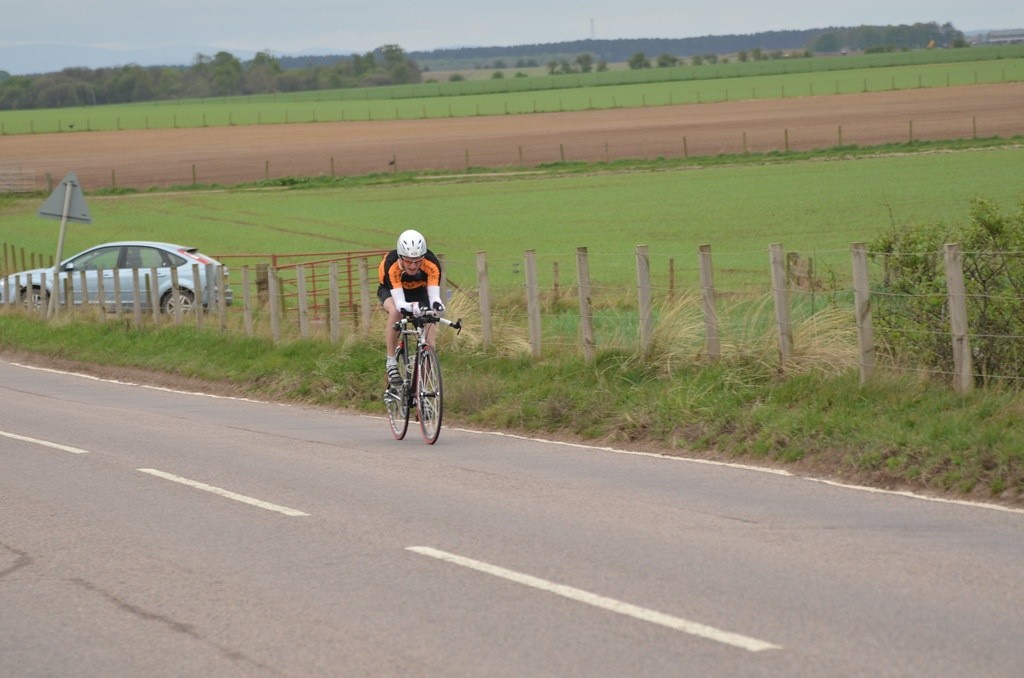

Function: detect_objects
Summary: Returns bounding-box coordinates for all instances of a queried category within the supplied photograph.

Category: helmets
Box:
[397,230,427,258]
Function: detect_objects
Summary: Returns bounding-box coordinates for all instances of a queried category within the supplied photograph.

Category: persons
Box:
[377,229,445,421]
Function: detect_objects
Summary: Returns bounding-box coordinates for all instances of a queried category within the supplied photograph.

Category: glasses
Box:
[401,255,425,264]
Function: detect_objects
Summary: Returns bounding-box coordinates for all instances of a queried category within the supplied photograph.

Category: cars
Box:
[0,240,233,319]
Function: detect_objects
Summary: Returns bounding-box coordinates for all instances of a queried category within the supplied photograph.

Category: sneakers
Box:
[385,361,404,387]
[420,397,432,420]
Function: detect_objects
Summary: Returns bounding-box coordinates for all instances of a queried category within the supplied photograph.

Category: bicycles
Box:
[382,303,462,444]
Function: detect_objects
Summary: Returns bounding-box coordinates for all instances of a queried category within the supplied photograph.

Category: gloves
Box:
[389,288,414,313]
[426,285,445,316]
